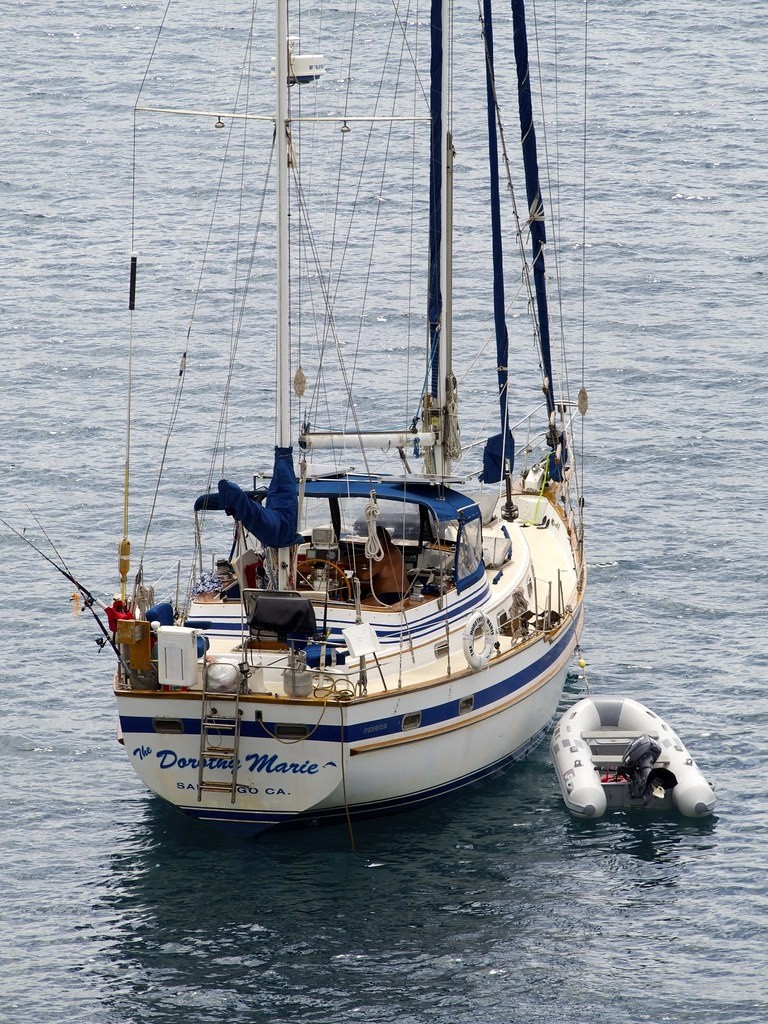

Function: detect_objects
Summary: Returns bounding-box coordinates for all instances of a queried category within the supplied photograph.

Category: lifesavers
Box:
[462,612,496,668]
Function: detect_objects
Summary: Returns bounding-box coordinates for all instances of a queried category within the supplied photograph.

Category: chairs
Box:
[232,549,257,590]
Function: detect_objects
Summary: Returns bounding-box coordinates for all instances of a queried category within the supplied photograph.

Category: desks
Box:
[339,536,428,569]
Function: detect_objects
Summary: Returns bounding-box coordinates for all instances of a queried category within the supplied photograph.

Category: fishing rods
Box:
[24,503,133,677]
[0,518,107,610]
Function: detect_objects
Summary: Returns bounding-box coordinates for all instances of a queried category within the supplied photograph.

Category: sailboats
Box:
[0,2,583,827]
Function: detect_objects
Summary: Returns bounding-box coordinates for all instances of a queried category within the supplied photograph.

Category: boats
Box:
[550,692,717,817]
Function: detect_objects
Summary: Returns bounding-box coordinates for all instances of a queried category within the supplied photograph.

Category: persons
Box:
[359,526,410,606]
